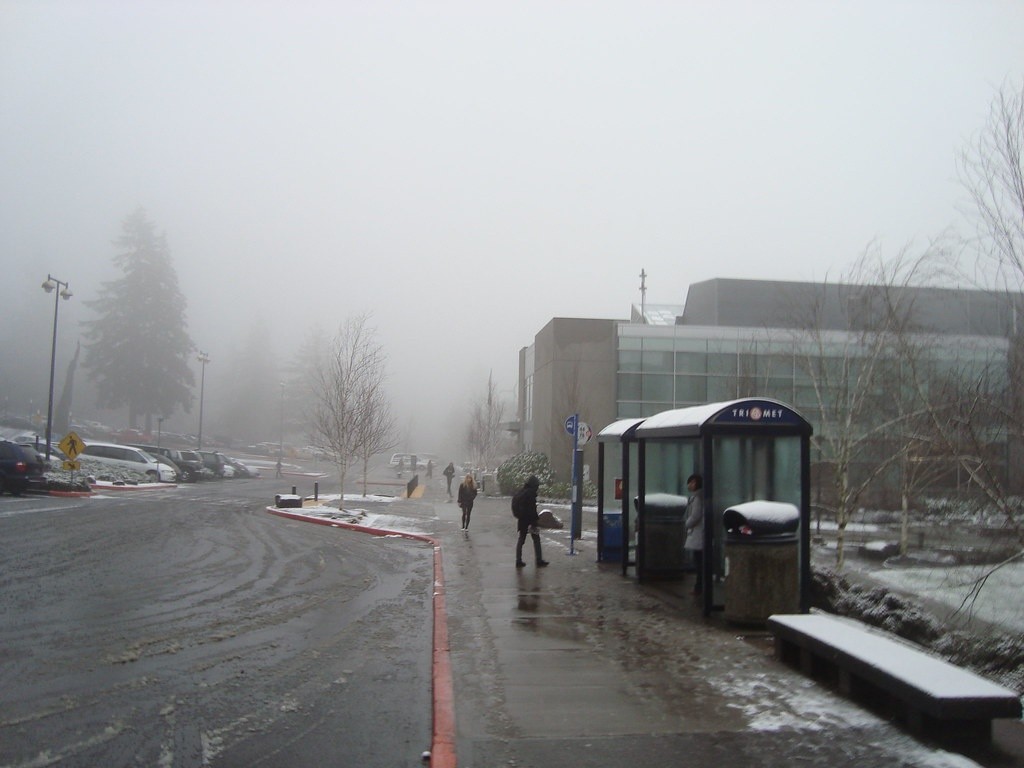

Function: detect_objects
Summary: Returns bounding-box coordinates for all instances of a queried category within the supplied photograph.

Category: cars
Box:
[229,457,249,477]
[7,414,234,481]
[239,459,258,477]
[388,448,436,470]
[245,441,337,458]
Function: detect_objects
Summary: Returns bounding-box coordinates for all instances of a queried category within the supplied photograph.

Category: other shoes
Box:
[516,562,526,567]
[536,561,549,567]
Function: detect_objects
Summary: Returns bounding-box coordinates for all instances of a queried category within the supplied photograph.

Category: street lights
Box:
[279,380,285,457]
[42,274,72,455]
[197,351,209,450]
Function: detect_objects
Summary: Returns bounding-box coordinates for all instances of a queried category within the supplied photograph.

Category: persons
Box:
[445,463,455,491]
[425,460,433,480]
[276,462,283,478]
[399,459,404,471]
[511,476,550,568]
[681,474,705,594]
[457,474,477,532]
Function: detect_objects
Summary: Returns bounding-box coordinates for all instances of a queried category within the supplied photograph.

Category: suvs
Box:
[0,440,48,494]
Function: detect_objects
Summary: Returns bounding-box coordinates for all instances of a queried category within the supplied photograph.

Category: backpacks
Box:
[512,488,531,519]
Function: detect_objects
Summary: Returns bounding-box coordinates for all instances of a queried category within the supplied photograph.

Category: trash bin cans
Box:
[723,502,801,630]
[632,493,689,579]
